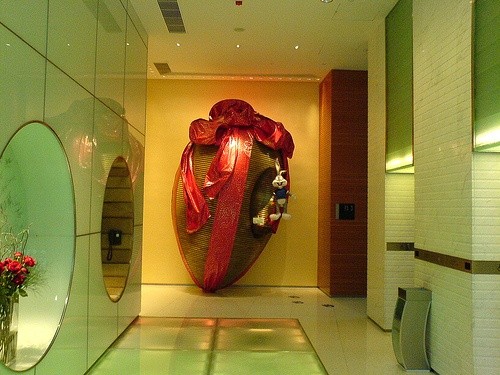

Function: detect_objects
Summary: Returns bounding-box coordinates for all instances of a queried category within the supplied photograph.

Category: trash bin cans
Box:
[390,286,431,373]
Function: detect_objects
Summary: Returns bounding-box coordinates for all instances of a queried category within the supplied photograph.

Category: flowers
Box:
[2,251,37,362]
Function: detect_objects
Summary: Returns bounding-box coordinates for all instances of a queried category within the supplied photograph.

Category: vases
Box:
[1,291,19,365]
